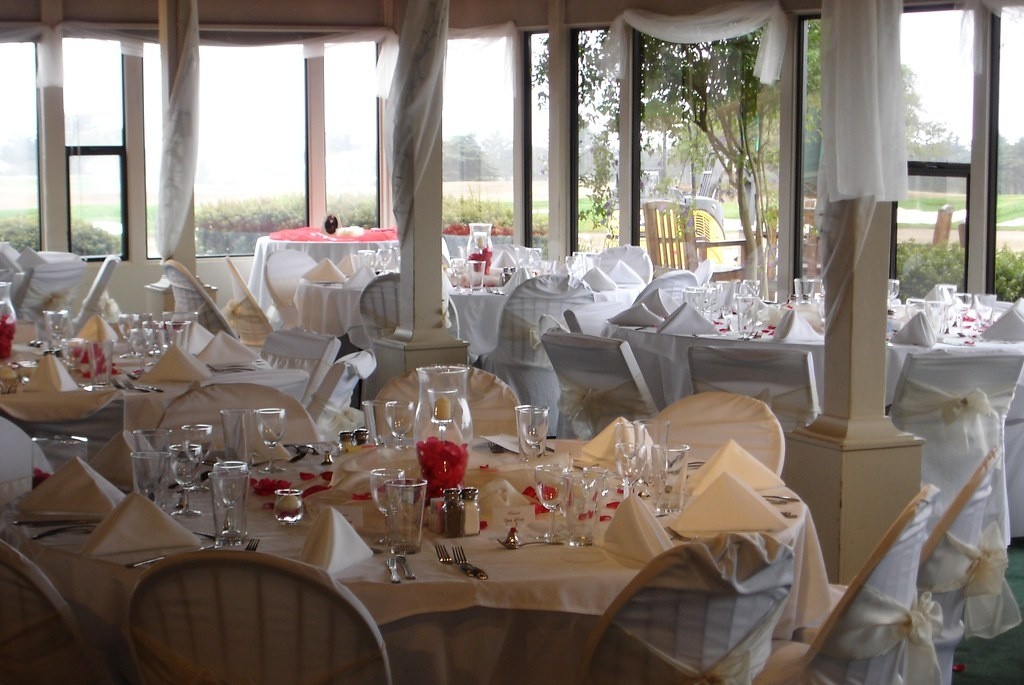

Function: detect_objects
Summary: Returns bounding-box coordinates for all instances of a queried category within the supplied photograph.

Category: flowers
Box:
[0,314,16,360]
[75,342,119,379]
[248,475,291,496]
[468,248,493,277]
[416,439,469,500]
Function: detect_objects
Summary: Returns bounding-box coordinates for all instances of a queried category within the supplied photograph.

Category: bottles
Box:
[440,488,464,538]
[413,365,473,459]
[460,487,482,537]
[467,222,493,259]
[1,282,17,359]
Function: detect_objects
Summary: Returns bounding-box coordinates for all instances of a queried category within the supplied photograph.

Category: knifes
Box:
[386,558,401,583]
[126,545,215,568]
[396,555,416,580]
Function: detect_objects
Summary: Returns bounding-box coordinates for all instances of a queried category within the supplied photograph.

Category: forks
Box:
[436,545,474,576]
[110,378,126,390]
[488,442,503,453]
[245,538,261,552]
[452,545,488,580]
[121,379,151,393]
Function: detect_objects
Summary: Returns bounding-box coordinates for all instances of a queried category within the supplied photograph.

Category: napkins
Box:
[0,251,1024,575]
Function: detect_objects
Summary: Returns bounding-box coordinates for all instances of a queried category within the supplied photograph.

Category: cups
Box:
[31,435,88,488]
[682,279,998,339]
[128,400,690,555]
[359,231,596,293]
[1,281,191,391]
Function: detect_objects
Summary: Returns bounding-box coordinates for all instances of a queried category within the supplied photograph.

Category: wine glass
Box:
[888,279,900,318]
[534,464,573,544]
[180,423,212,492]
[377,249,392,275]
[451,259,466,292]
[384,401,415,451]
[614,423,647,500]
[255,408,285,474]
[117,313,142,359]
[131,328,153,376]
[168,443,204,517]
[369,468,405,547]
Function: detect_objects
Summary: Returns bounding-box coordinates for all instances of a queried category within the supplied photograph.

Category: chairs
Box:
[754,482,937,685]
[0,541,118,685]
[123,547,392,685]
[643,202,744,286]
[796,446,999,684]
[930,205,953,247]
[574,530,796,685]
[0,245,1024,536]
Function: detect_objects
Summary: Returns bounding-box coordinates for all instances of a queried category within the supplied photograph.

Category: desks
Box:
[603,302,1024,537]
[295,261,645,357]
[254,227,451,319]
[0,439,830,685]
[0,338,311,471]
